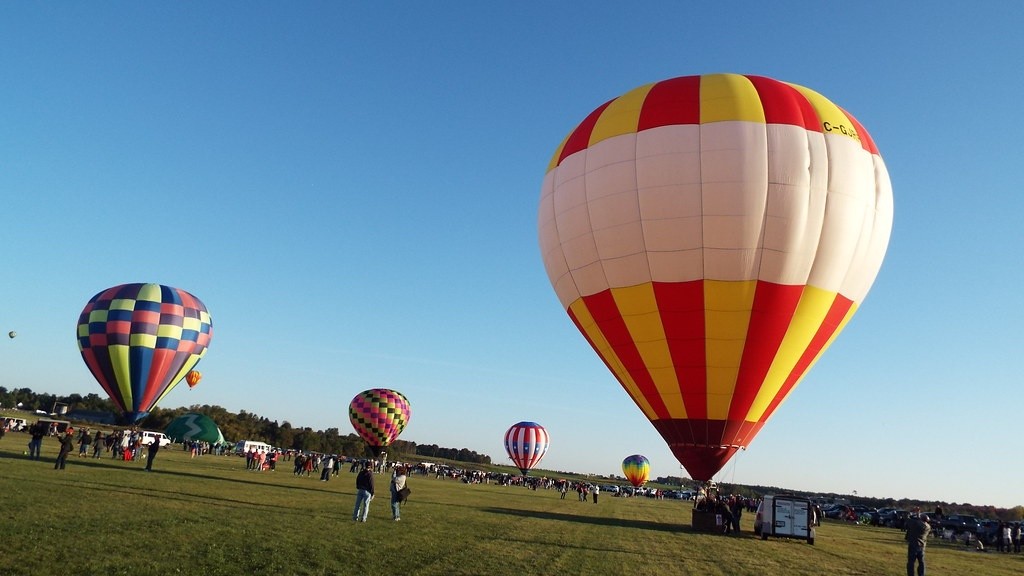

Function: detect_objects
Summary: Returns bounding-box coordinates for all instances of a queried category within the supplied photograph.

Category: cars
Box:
[822,501,983,533]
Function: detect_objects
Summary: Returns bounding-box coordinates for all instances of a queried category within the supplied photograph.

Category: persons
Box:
[484,472,524,486]
[702,493,743,535]
[974,520,1022,553]
[527,476,571,499]
[349,458,382,473]
[77,428,142,460]
[614,486,638,498]
[144,434,160,472]
[645,488,663,500]
[293,451,342,482]
[352,462,375,522]
[905,507,931,576]
[182,438,231,458]
[404,461,445,480]
[447,468,484,484]
[390,467,407,521]
[245,448,292,471]
[0,417,73,470]
[741,496,764,512]
[571,481,599,504]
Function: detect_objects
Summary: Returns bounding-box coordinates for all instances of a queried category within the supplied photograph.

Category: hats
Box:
[67,428,74,434]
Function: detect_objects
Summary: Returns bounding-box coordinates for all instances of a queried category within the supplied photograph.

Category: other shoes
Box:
[353,517,358,521]
[361,518,367,522]
[394,518,401,522]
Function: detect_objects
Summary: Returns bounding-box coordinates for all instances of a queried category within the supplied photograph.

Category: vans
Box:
[137,431,171,450]
[235,440,269,458]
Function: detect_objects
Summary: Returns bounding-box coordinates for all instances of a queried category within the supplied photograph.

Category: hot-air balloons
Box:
[348,388,412,461]
[77,282,213,426]
[622,454,650,488]
[8,331,17,338]
[186,370,202,390]
[538,73,893,495]
[502,420,550,482]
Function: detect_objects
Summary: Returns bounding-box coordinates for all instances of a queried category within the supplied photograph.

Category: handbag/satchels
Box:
[395,487,413,505]
